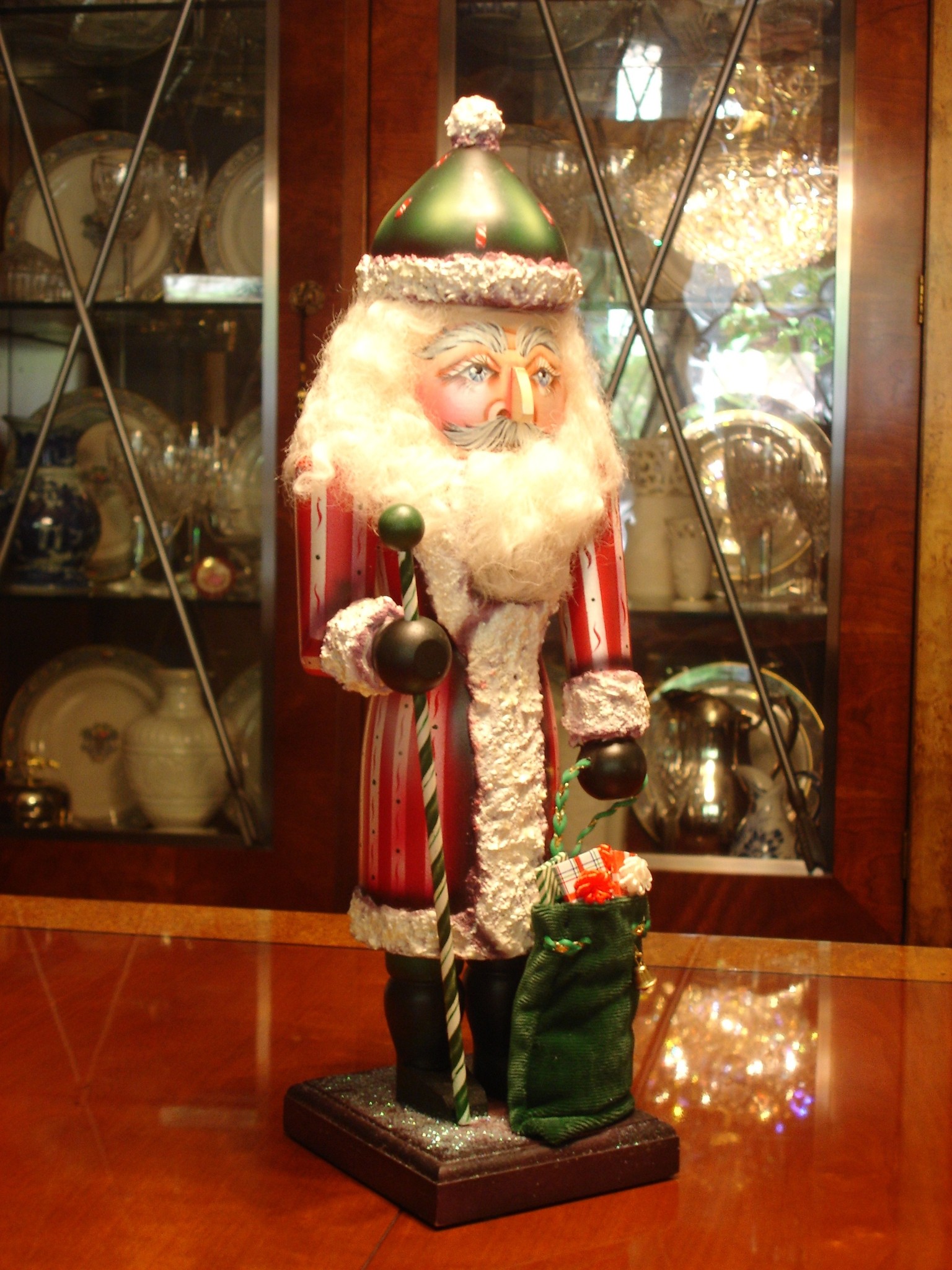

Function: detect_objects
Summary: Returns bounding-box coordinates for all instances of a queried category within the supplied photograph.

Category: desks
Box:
[0,887,952,1270]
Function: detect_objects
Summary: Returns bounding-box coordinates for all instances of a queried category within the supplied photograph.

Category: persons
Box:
[277,92,651,1120]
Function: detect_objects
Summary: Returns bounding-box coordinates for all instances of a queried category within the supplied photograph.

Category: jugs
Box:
[657,687,799,854]
[730,764,797,859]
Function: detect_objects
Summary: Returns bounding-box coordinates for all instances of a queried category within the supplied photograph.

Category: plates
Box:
[2,389,187,581]
[218,406,263,536]
[214,661,265,840]
[199,134,263,277]
[4,130,199,303]
[658,393,832,598]
[633,661,824,859]
[2,646,172,833]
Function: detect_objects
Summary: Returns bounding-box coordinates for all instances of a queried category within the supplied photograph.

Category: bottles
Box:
[1,414,102,583]
[120,669,228,835]
[613,439,701,612]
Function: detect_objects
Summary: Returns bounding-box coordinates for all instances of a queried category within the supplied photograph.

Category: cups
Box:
[526,143,591,229]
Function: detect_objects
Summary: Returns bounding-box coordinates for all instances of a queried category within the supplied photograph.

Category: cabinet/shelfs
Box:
[1,0,938,946]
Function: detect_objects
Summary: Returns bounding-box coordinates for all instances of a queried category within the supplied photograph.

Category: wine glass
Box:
[788,437,832,613]
[159,150,205,273]
[590,147,644,302]
[92,155,156,302]
[108,422,240,596]
[666,515,715,610]
[723,417,794,603]
